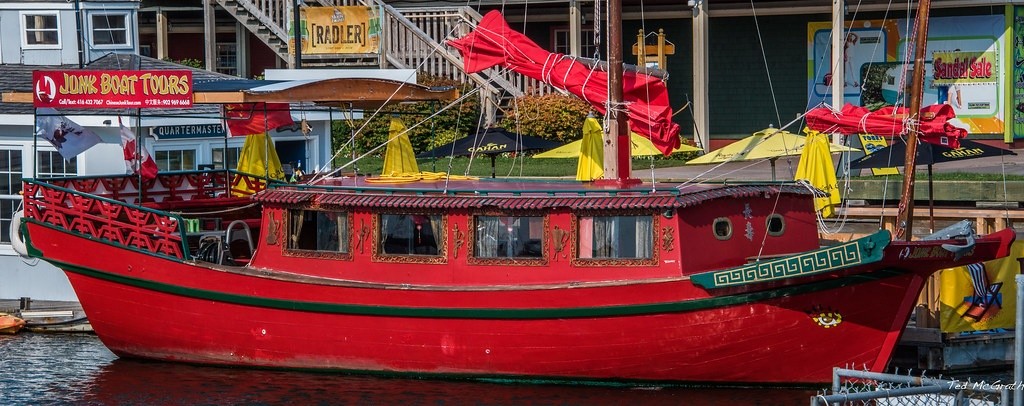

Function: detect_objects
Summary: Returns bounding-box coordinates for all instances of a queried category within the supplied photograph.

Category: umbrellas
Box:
[382,112,419,175]
[415,122,562,179]
[533,124,703,159]
[794,125,841,218]
[685,124,861,181]
[576,110,604,182]
[231,134,285,198]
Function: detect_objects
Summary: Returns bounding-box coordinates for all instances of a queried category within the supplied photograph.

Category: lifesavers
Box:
[9,209,28,258]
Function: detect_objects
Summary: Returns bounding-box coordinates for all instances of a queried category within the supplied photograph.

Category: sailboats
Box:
[8,0,1018,388]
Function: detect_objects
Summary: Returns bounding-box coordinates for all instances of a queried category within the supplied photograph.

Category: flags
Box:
[33,107,102,163]
[118,117,158,183]
[220,103,294,137]
